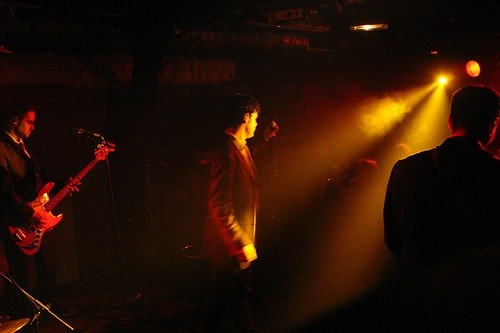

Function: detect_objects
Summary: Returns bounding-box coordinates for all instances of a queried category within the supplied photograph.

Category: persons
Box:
[0,99,82,332]
[382,84,500,333]
[203,93,282,333]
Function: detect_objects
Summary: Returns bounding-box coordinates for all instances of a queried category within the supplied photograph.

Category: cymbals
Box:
[0,317,31,333]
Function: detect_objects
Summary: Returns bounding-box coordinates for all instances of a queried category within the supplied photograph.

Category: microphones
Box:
[269,121,277,130]
[73,127,105,142]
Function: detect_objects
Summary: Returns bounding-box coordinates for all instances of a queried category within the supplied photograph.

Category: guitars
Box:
[8,140,116,258]
